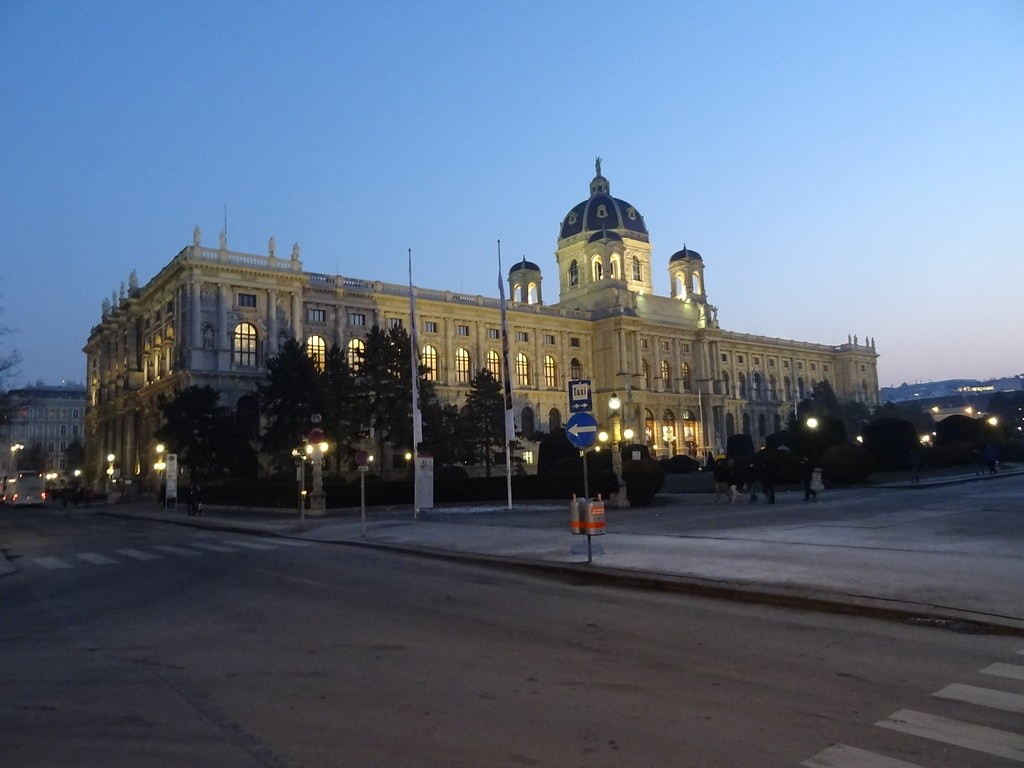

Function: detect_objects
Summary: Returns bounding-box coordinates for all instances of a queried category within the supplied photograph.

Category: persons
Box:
[801,453,819,502]
[711,458,778,505]
[85,487,93,504]
[972,439,998,476]
[186,482,202,517]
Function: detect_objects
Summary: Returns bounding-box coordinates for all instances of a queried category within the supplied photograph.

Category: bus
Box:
[0,470,46,506]
